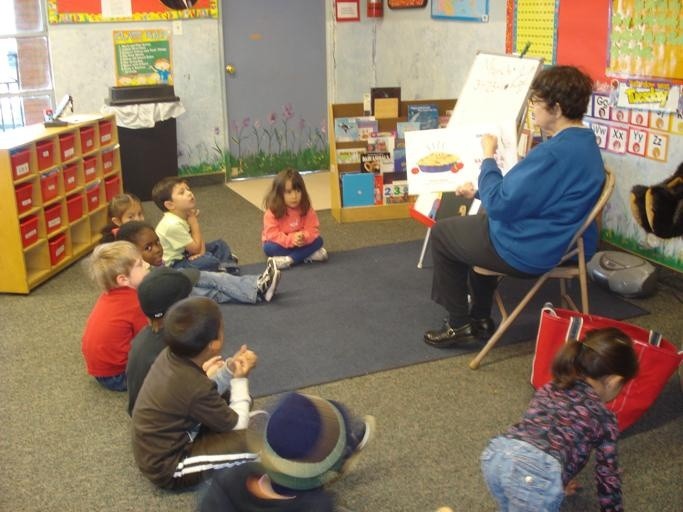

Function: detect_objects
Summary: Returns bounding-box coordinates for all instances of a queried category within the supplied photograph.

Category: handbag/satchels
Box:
[530,300,681,431]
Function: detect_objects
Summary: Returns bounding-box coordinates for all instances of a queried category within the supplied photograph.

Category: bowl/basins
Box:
[418,162,458,173]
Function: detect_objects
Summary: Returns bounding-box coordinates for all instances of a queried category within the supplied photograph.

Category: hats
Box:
[259,390,377,489]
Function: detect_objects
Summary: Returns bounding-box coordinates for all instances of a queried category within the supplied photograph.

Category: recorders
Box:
[585,249,660,299]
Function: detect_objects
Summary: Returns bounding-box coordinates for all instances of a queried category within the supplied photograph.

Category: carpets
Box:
[223,169,331,214]
[215,237,652,399]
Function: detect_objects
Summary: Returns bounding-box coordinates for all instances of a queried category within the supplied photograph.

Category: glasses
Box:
[529,92,545,105]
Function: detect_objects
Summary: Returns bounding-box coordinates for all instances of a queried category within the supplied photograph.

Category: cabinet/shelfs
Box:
[0,112,124,298]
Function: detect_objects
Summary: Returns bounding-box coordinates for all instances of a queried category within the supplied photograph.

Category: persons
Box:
[105,194,280,304]
[437,326,639,512]
[424,65,607,349]
[152,176,241,277]
[131,295,269,492]
[81,241,150,393]
[98,220,164,266]
[261,168,329,269]
[126,261,254,420]
[194,391,376,512]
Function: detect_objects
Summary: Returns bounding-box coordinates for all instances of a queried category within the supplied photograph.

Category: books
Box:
[334,87,452,207]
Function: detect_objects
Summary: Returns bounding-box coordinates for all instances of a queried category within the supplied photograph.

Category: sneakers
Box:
[257,258,281,301]
[266,256,291,270]
[306,247,330,262]
[216,250,239,272]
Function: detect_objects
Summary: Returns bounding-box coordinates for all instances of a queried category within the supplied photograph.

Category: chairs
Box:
[468,162,616,370]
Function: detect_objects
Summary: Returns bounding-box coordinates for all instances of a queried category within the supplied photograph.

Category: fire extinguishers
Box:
[366,0,383,18]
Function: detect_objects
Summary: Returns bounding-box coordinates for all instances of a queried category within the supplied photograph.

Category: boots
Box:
[472,314,496,342]
[424,319,479,349]
